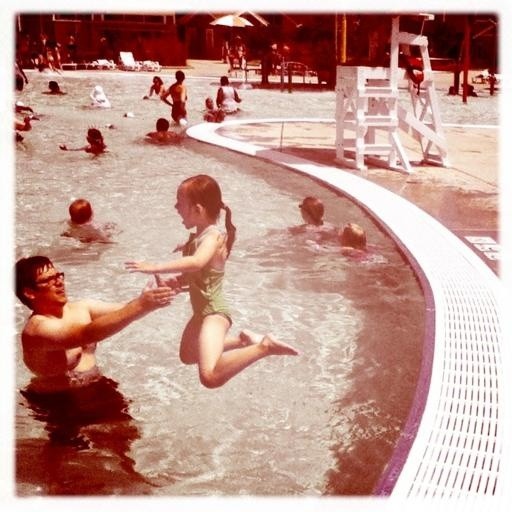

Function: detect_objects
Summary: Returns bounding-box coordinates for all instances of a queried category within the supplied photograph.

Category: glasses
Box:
[37,272,64,285]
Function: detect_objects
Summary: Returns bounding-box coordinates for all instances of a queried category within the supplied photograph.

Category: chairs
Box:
[225,56,262,80]
[61,52,163,72]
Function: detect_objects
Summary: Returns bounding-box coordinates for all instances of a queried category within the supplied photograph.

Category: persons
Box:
[461,82,478,97]
[124,173,299,390]
[327,220,371,257]
[486,72,497,95]
[62,199,111,249]
[16,30,294,157]
[282,194,332,240]
[15,252,185,387]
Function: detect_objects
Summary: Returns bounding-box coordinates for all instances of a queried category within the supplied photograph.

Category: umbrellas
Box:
[209,15,255,37]
